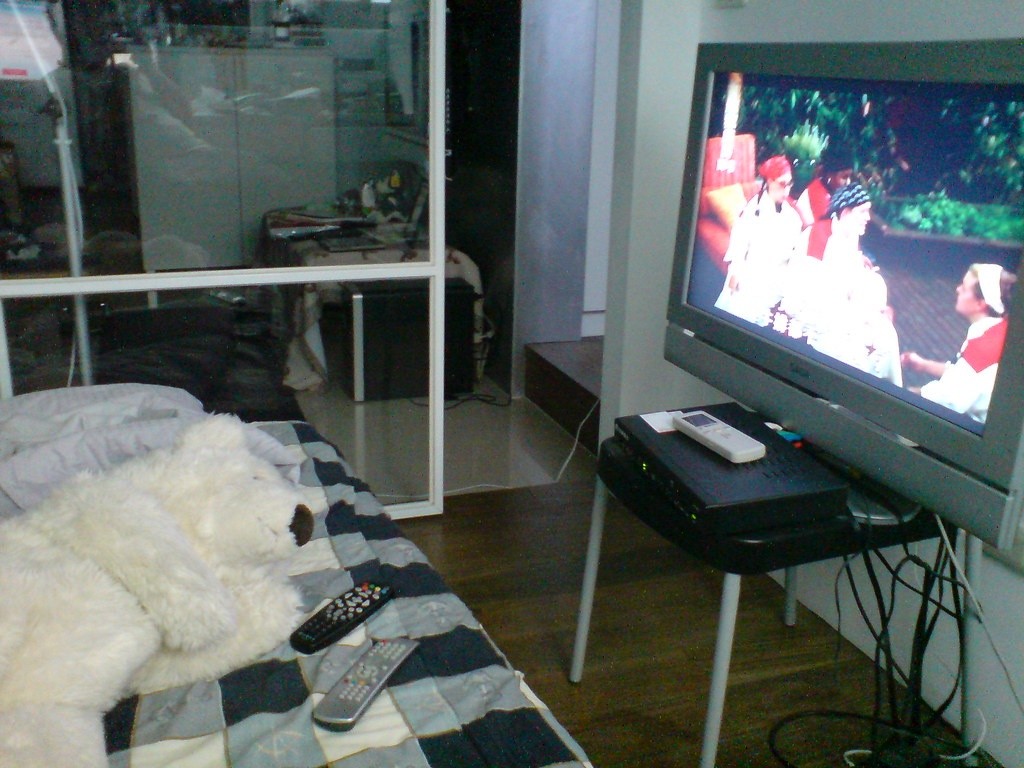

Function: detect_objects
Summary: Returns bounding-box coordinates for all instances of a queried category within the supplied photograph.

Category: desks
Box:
[265,202,487,396]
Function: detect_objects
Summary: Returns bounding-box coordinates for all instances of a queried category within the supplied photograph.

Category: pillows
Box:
[0,382,204,460]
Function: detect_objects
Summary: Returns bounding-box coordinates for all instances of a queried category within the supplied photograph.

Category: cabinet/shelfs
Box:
[113,42,342,315]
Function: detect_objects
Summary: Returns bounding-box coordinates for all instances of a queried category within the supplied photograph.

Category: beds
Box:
[0,382,597,768]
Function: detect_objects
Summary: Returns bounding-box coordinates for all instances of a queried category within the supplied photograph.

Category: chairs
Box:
[568,430,984,768]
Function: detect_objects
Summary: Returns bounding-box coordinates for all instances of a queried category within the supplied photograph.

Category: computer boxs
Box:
[343,275,476,401]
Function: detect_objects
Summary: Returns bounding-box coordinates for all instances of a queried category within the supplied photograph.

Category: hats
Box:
[820,183,871,220]
[821,141,854,171]
[759,156,791,181]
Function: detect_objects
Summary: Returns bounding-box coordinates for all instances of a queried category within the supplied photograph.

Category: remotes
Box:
[310,638,421,732]
[673,410,766,465]
[289,582,394,654]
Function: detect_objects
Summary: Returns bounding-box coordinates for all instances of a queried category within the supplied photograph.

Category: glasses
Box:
[773,180,794,188]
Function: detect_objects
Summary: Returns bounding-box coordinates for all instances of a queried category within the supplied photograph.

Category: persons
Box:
[901,261,1018,423]
[806,181,905,389]
[716,156,804,329]
[795,145,855,265]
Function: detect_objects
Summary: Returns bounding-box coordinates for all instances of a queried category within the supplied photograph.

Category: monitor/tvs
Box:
[664,40,1024,551]
